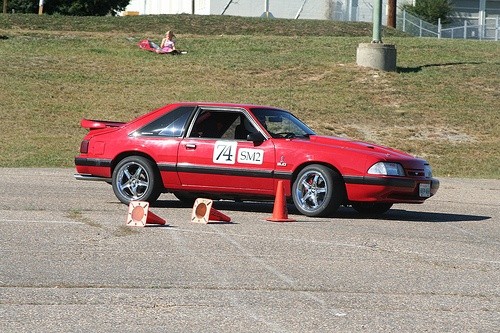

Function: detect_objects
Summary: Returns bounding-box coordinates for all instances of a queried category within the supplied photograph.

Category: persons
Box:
[241,112,278,140]
[160,30,176,54]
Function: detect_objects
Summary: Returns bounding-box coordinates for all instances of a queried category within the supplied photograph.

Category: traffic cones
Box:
[191,198,232,224]
[126,201,167,228]
[266,181,296,221]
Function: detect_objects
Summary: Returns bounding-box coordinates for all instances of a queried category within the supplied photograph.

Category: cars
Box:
[75,102,441,218]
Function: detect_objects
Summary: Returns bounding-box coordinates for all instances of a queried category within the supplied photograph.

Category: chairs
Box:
[234,114,250,138]
